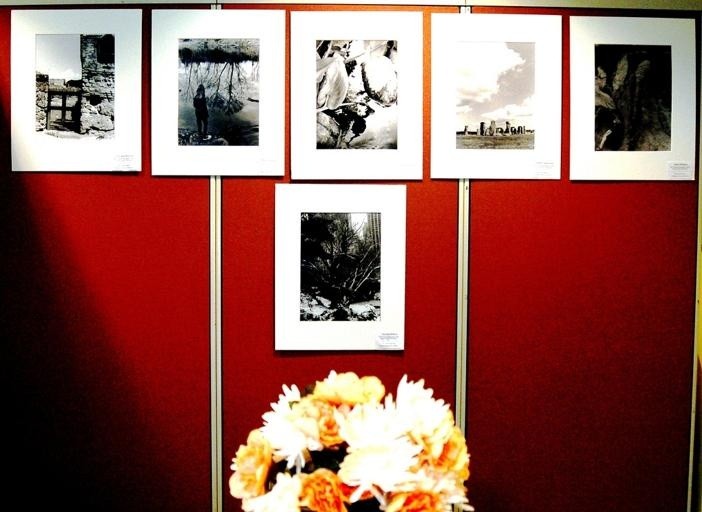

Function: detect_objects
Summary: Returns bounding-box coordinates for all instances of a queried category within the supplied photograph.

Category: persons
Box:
[192,85,213,141]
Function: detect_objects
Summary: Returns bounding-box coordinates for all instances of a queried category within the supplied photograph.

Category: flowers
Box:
[229,366,474,512]
